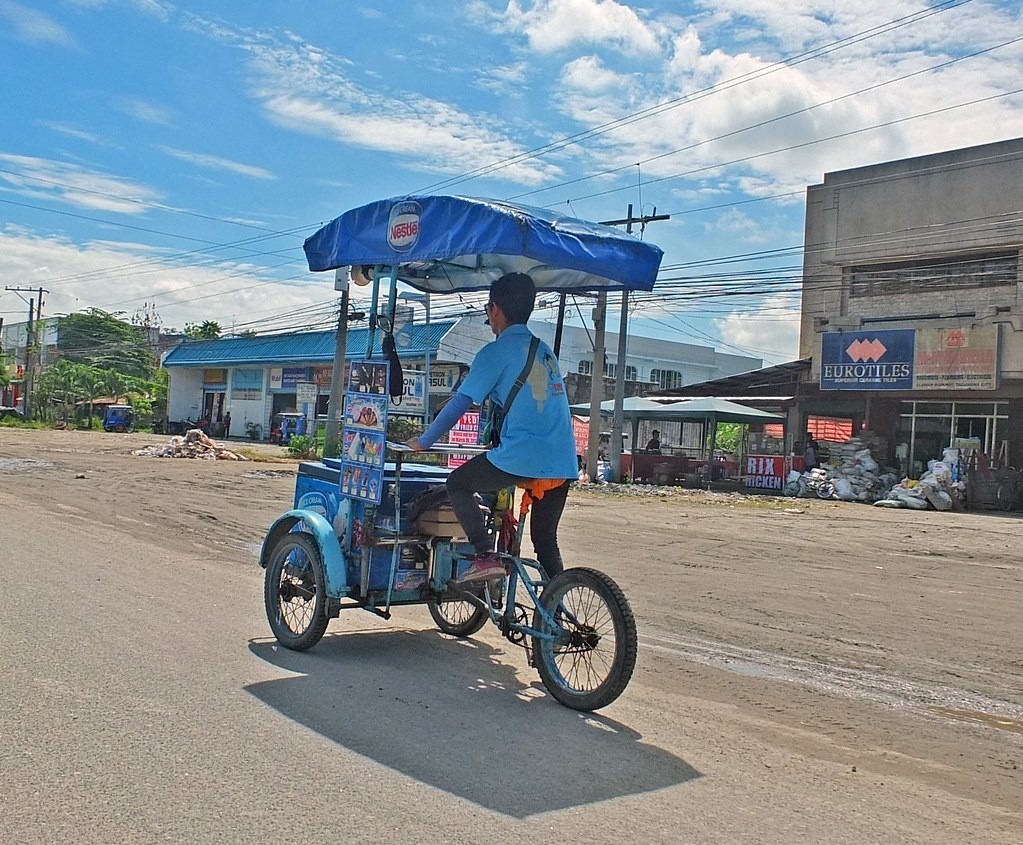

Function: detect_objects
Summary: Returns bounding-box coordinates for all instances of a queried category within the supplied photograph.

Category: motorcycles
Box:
[150,417,164,435]
[182,413,213,437]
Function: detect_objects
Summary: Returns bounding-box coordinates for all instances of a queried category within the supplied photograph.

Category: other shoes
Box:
[456,551,506,584]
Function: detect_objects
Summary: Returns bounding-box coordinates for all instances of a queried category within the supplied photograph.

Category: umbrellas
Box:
[569,395,784,493]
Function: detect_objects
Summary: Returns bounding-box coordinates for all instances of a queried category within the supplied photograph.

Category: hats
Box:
[652,429,660,433]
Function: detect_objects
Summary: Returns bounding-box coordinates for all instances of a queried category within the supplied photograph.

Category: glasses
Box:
[484,303,492,314]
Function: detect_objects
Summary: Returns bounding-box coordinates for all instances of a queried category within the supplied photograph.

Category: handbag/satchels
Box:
[488,404,503,448]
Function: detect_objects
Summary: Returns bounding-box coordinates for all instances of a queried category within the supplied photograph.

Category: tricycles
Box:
[258,194,663,712]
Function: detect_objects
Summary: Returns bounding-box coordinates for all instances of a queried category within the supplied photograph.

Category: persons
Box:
[295,415,305,435]
[272,416,288,438]
[224,411,231,438]
[597,436,609,461]
[641,430,660,484]
[402,271,579,666]
[205,408,216,437]
[804,442,815,472]
[807,432,821,467]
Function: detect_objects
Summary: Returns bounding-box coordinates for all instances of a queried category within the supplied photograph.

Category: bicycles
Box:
[784,465,836,500]
[997,466,1023,511]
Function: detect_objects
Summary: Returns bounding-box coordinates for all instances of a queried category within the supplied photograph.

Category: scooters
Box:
[102,405,137,433]
[270,413,304,446]
